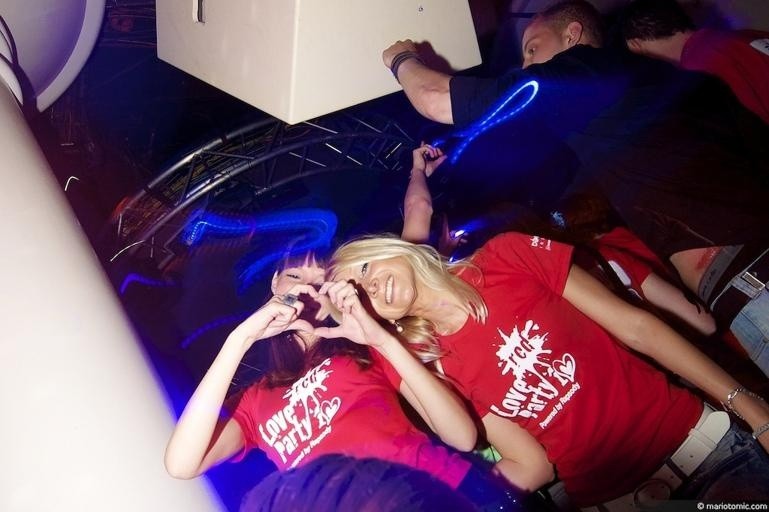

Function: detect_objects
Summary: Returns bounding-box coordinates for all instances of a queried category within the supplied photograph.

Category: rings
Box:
[282,294,299,305]
[354,288,359,296]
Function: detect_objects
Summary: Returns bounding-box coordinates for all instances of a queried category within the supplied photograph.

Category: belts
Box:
[711,252,769,328]
[574,399,731,512]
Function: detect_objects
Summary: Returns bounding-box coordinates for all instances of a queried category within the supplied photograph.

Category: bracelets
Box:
[721,385,765,421]
[389,51,427,85]
[751,422,769,441]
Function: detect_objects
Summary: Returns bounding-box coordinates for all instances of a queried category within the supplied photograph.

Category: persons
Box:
[322,233,769,511]
[381,0,768,380]
[564,226,753,363]
[163,232,529,511]
[401,139,471,263]
[604,0,769,125]
